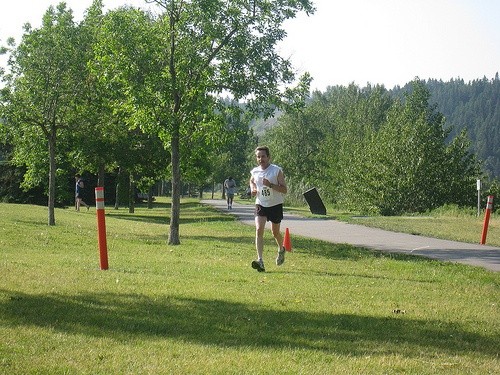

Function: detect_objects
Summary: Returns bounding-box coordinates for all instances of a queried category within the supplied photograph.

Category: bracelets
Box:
[270,182,273,188]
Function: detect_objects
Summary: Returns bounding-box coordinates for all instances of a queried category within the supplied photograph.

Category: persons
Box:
[74,173,90,211]
[248,146,288,272]
[223,175,237,212]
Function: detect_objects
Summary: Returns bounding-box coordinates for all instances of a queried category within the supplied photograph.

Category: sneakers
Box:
[276,246,285,266]
[252,259,265,273]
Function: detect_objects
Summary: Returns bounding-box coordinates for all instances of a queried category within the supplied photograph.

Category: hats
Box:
[74,173,82,178]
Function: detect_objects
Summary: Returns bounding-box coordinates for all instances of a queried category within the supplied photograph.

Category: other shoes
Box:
[76,209,79,211]
[87,205,89,210]
